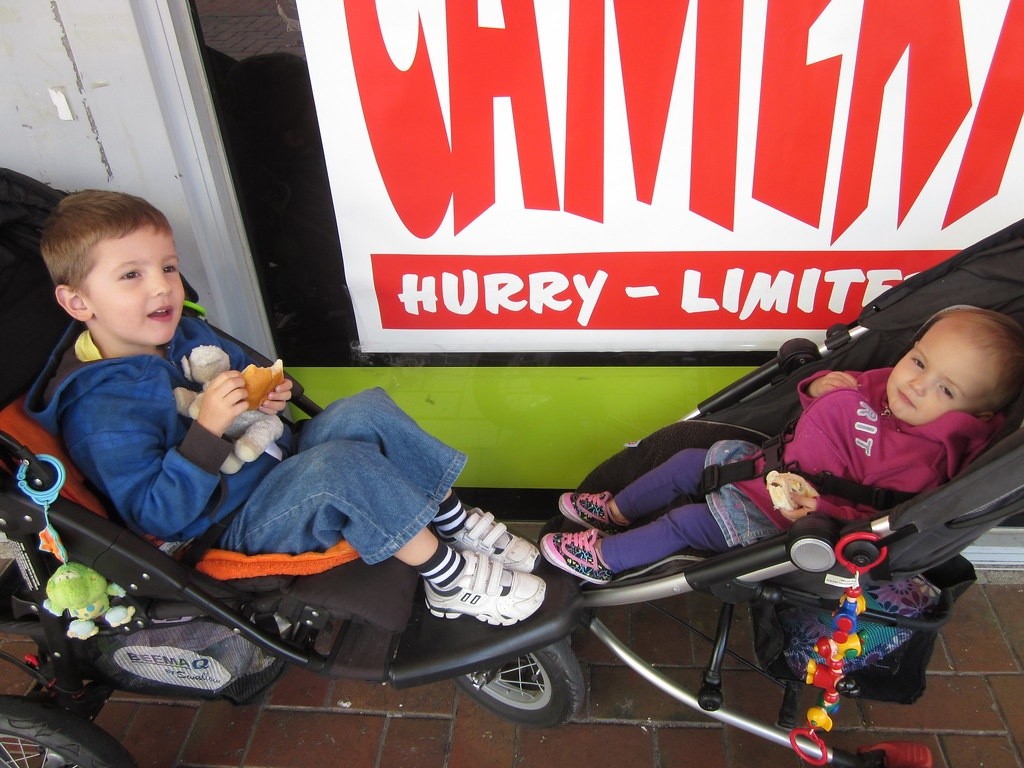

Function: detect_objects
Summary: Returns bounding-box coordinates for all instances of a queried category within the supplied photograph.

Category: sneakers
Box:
[435,507,542,573]
[559,491,632,537]
[423,550,545,626]
[540,528,612,585]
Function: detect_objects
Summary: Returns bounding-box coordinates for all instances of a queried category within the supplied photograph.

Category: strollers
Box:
[534,217,1023,767]
[0,167,585,768]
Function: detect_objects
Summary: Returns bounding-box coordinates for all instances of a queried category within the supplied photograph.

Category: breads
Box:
[241,358,284,412]
[765,469,820,513]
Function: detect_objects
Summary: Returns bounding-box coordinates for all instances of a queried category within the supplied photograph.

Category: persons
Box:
[39,190,548,626]
[540,305,1024,585]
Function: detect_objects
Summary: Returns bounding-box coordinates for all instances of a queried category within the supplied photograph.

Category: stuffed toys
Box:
[31,505,136,640]
[173,345,283,475]
